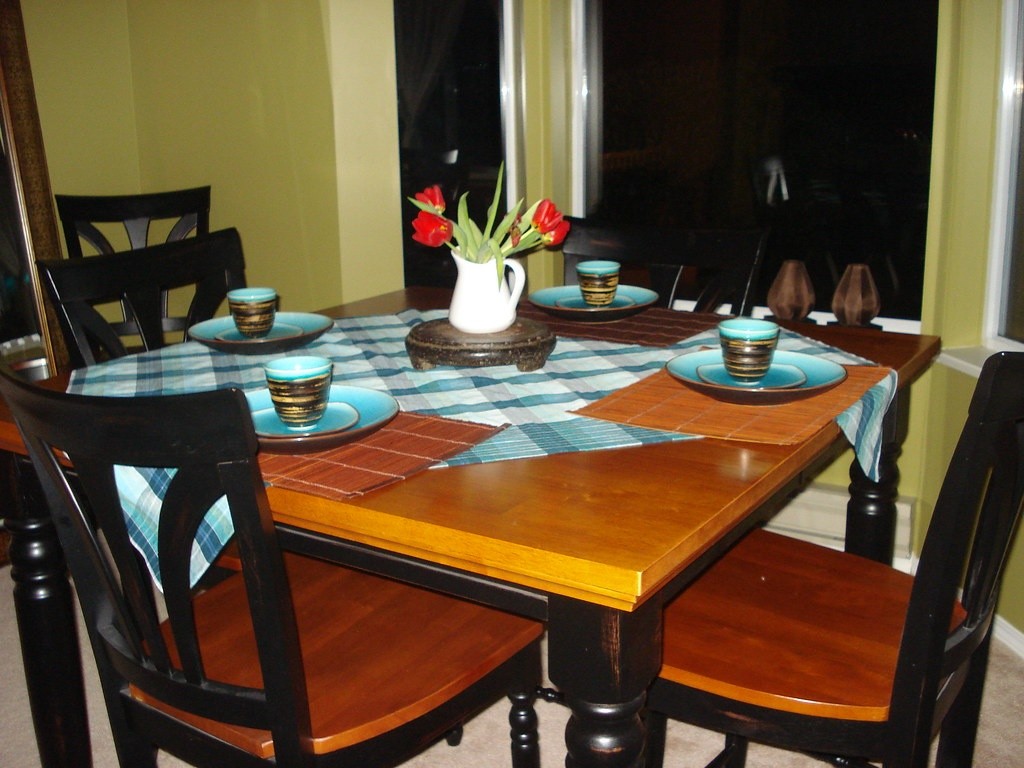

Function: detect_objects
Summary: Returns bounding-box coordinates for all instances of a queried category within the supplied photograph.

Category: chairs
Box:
[0,372,541,768]
[598,349,1024,768]
[33,228,250,584]
[556,216,768,317]
[52,186,212,353]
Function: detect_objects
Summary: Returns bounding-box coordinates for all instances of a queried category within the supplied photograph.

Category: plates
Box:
[665,348,848,406]
[245,385,400,455]
[527,284,659,322]
[187,311,334,356]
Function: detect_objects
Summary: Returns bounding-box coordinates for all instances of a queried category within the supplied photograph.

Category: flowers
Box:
[403,160,569,281]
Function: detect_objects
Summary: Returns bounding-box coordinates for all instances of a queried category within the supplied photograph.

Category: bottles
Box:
[766,259,815,320]
[831,263,882,326]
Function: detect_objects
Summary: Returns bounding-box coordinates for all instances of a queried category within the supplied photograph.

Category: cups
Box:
[575,260,620,306]
[226,288,278,337]
[716,317,781,386]
[263,355,333,431]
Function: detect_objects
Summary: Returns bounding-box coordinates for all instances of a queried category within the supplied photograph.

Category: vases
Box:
[446,242,525,336]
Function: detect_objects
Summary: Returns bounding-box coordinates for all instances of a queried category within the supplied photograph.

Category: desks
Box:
[0,287,942,768]
